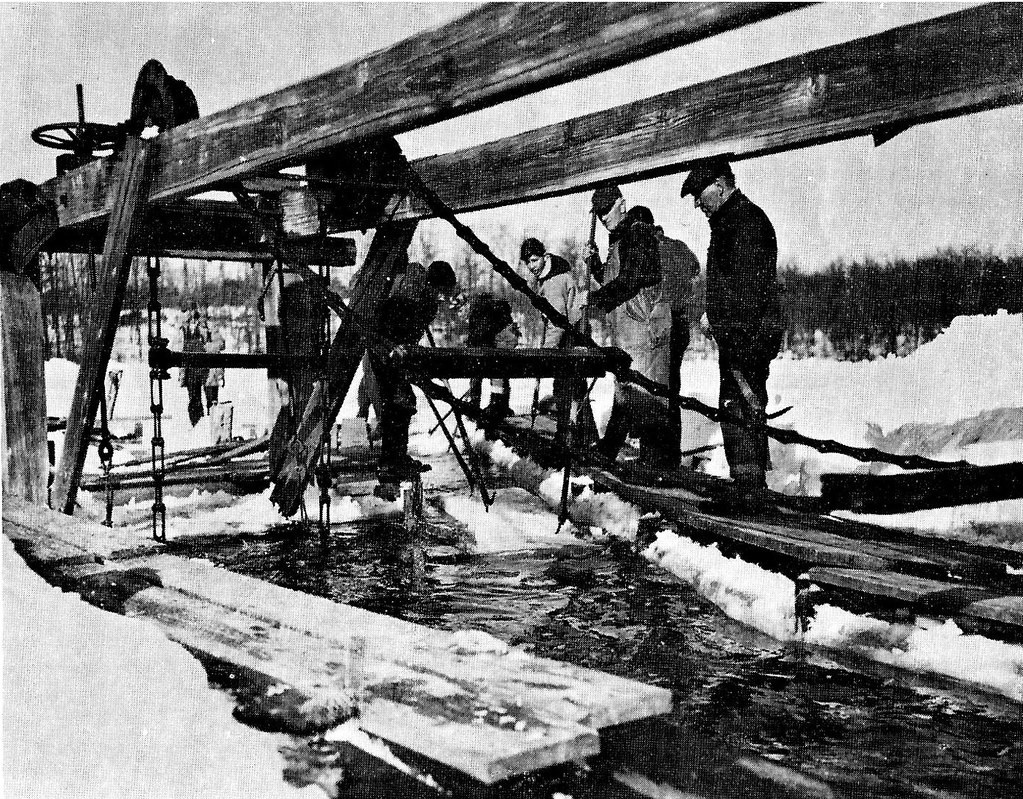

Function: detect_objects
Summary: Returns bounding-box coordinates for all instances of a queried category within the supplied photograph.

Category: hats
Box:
[588,185,622,215]
[427,260,457,287]
[680,161,732,200]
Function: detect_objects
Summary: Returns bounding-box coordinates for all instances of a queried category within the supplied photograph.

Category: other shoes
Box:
[379,455,420,467]
[591,442,619,460]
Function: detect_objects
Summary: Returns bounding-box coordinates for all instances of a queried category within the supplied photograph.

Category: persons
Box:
[203,339,225,415]
[519,238,600,441]
[450,292,520,416]
[579,187,700,481]
[356,261,457,478]
[178,332,207,425]
[680,160,784,485]
